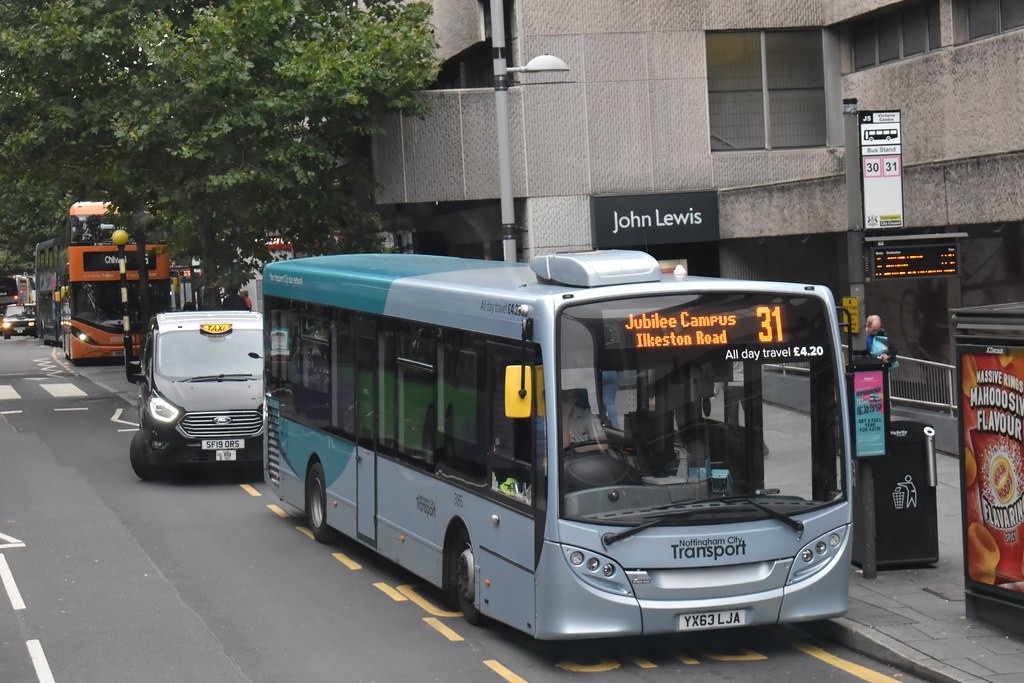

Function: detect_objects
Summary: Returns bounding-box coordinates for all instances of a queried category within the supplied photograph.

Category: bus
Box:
[0,273,36,316]
[262,248,857,643]
[35,201,182,367]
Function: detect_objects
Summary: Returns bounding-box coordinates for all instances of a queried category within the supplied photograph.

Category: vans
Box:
[126,311,262,482]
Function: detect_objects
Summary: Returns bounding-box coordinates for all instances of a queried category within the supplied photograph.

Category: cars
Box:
[0,302,36,339]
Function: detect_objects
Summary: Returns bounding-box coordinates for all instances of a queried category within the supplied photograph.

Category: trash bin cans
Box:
[852,420,939,569]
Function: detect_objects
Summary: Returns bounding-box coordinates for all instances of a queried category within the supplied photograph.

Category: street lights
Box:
[494,51,571,261]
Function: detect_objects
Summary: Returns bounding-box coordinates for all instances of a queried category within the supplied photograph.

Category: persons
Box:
[865,315,898,367]
[223,287,252,310]
[561,388,609,453]
